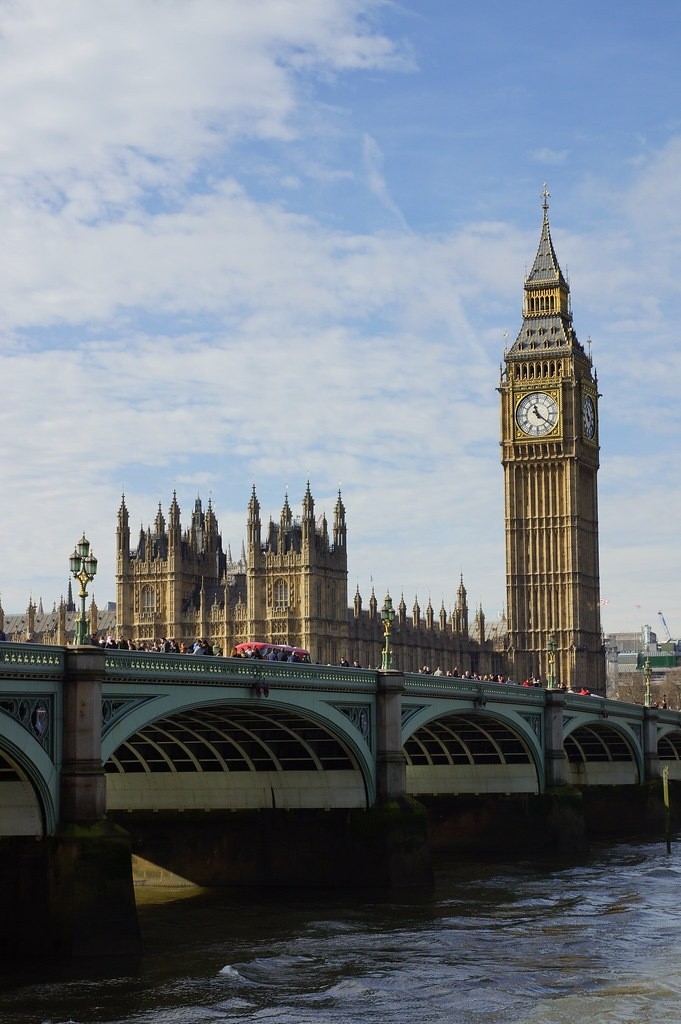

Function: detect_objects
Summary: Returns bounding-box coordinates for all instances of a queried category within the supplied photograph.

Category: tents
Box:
[235,641,308,660]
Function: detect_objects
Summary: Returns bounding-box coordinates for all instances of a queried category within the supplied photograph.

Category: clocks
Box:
[581,390,597,444]
[514,389,560,440]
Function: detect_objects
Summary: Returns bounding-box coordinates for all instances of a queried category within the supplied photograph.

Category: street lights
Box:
[380,589,397,669]
[644,656,653,706]
[547,632,558,689]
[69,530,98,647]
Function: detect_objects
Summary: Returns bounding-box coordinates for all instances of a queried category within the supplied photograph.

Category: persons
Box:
[660,699,667,710]
[230,644,311,664]
[0,626,7,662]
[557,683,591,696]
[90,633,223,656]
[25,633,36,643]
[418,665,542,688]
[339,656,363,668]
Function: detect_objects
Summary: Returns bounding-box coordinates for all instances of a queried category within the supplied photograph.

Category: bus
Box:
[235,641,310,663]
